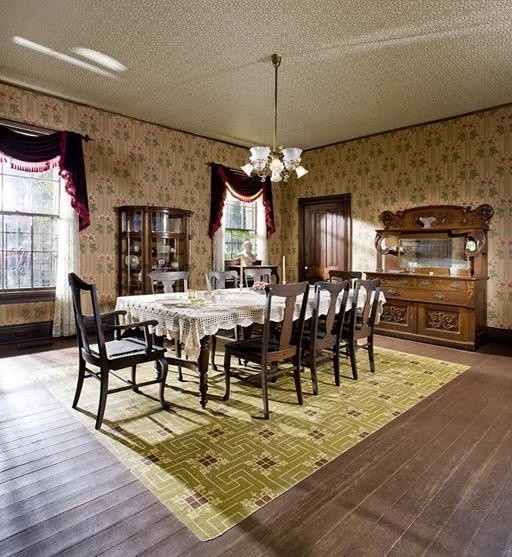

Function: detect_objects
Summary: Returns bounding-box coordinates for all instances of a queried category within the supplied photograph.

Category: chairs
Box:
[244,269,272,287]
[300,281,352,396]
[206,270,238,290]
[148,271,189,294]
[328,270,362,283]
[342,277,381,380]
[67,272,170,431]
[223,281,309,420]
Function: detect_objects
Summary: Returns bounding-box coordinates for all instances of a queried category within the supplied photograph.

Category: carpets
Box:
[41,331,472,542]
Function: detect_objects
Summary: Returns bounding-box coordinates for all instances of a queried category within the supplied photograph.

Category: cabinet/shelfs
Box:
[225,260,279,289]
[111,205,195,296]
[363,204,495,353]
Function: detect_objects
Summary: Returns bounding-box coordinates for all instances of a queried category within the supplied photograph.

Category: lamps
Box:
[240,53,309,183]
